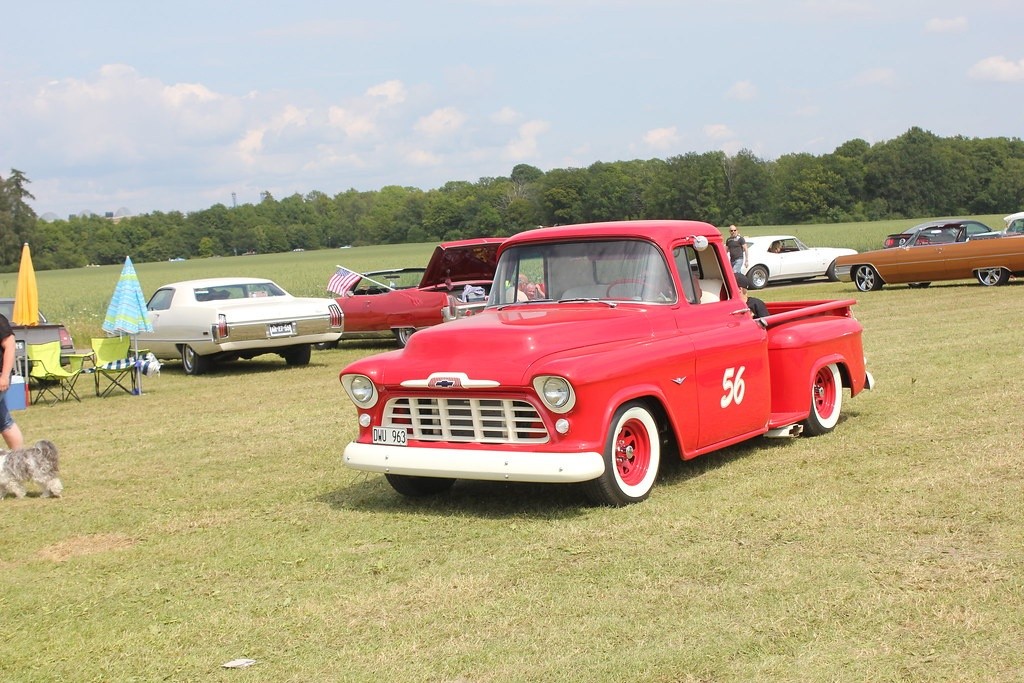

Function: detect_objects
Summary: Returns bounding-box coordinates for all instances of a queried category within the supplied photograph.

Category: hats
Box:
[734,273,749,289]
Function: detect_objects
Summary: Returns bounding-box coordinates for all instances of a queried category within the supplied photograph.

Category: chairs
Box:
[85,334,150,396]
[20,338,85,405]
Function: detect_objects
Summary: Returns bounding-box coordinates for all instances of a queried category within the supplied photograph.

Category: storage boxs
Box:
[6,374,27,412]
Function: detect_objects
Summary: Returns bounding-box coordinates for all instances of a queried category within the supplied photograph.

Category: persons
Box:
[771,241,781,253]
[725,224,748,273]
[506,274,546,303]
[733,273,770,319]
[0,313,24,451]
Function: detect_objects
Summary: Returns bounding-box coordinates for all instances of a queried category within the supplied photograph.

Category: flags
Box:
[326,268,361,296]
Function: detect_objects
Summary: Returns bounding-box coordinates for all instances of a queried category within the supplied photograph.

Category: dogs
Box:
[0,439,64,500]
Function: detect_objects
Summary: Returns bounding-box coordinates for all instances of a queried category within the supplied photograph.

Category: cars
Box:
[883,211,1024,250]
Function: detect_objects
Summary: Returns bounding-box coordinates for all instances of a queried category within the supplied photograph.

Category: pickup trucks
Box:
[338,218,874,507]
[0,299,76,374]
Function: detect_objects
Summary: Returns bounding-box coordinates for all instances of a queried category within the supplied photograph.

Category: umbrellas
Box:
[77,352,164,378]
[102,255,154,396]
[13,242,40,407]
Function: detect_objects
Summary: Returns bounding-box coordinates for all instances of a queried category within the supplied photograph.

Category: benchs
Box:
[697,276,724,302]
[559,280,658,301]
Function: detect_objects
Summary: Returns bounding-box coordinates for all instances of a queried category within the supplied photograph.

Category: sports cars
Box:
[688,236,862,291]
[316,236,530,349]
[834,225,1024,292]
[108,277,345,376]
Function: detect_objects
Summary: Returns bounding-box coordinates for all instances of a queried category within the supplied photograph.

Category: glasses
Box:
[730,230,736,232]
